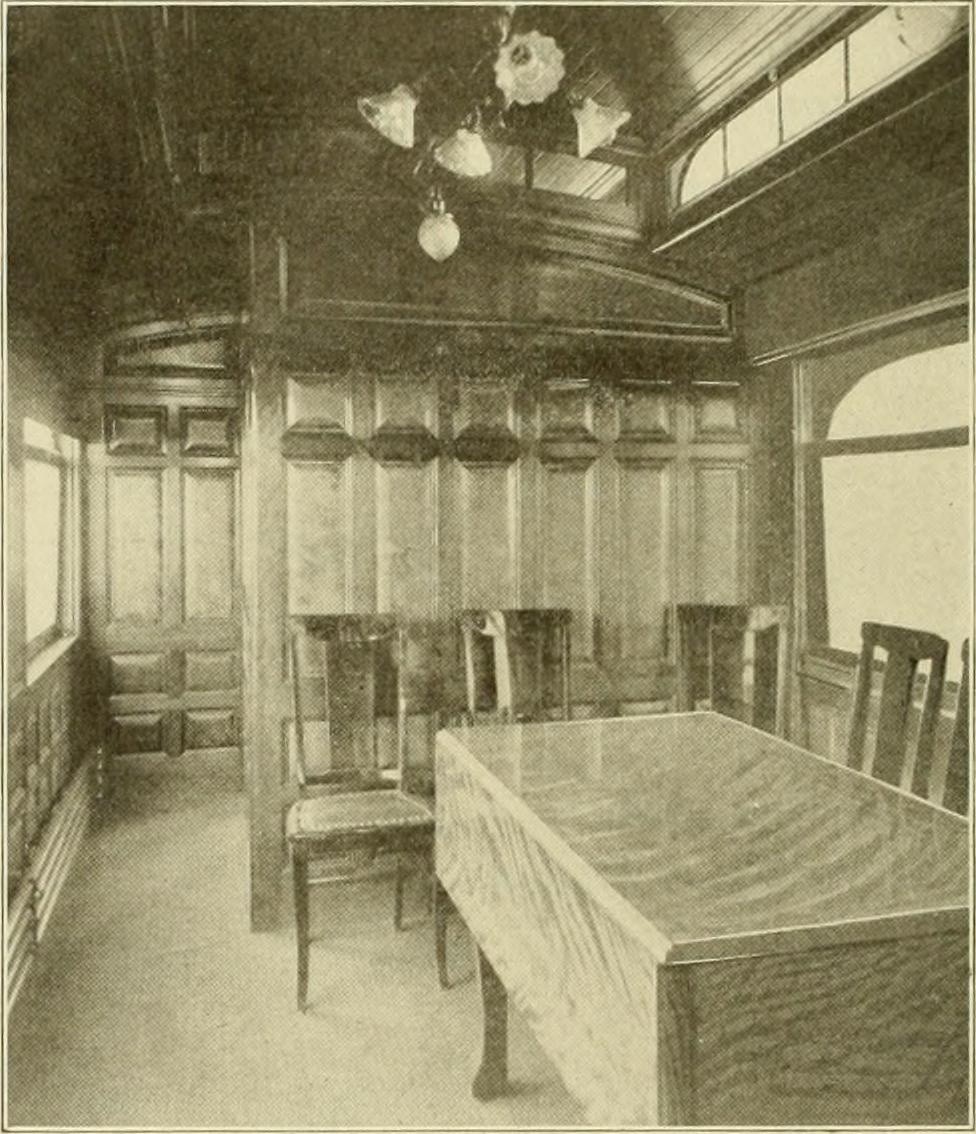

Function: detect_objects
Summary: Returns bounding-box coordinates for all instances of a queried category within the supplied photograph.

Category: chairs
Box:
[283,605,970,1008]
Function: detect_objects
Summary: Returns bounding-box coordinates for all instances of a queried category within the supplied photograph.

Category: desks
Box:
[433,710,969,1125]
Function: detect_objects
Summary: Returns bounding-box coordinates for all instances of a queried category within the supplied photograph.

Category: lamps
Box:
[358,6,631,266]
